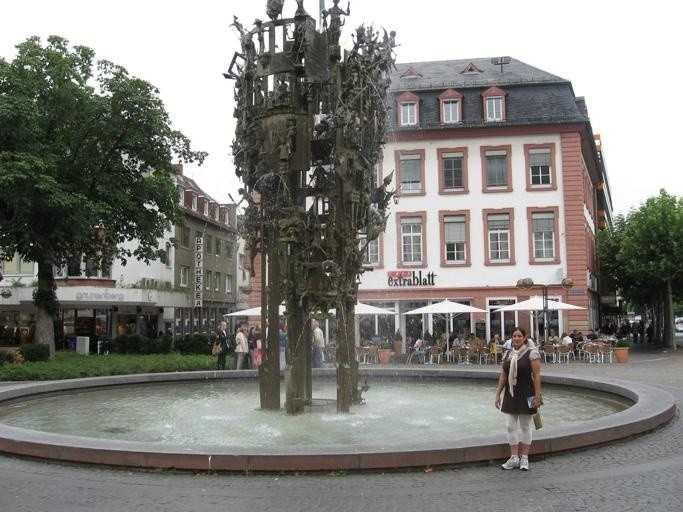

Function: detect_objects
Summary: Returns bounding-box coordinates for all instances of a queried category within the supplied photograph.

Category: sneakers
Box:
[502,456,529,471]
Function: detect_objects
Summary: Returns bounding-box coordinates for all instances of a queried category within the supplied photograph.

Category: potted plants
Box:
[378,328,401,364]
[612,339,629,363]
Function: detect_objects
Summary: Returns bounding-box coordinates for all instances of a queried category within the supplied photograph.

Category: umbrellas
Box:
[317,300,399,315]
[222,304,314,317]
[494,292,588,343]
[401,298,489,363]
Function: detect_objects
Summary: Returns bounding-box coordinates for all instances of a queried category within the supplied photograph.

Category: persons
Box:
[323,0,350,43]
[586,318,654,344]
[214,315,325,369]
[408,328,583,364]
[312,159,327,188]
[378,169,395,195]
[495,327,542,471]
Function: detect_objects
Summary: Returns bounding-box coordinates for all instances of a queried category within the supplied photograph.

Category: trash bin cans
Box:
[64,334,76,352]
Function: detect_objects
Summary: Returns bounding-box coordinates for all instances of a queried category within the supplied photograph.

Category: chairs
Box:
[407,334,612,365]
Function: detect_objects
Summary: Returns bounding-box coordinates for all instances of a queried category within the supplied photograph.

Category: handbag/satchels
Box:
[533,413,543,430]
[212,341,222,356]
